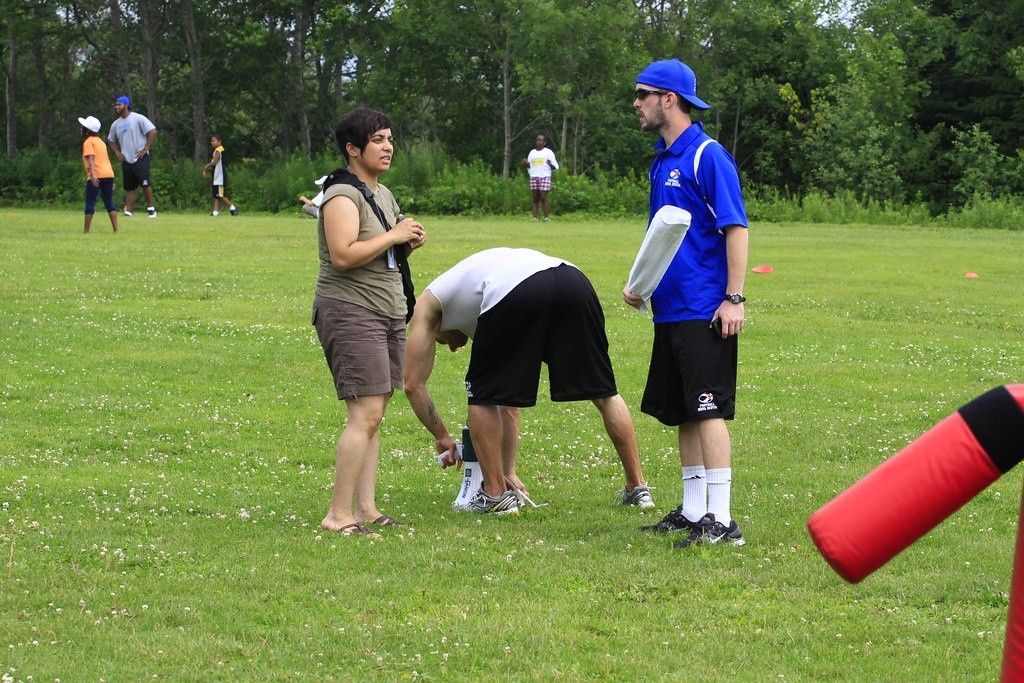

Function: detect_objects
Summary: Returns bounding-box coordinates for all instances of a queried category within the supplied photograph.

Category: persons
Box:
[311,108,427,540]
[202,134,238,215]
[298,175,328,218]
[622,59,749,548]
[404,246,654,514]
[107,96,156,218]
[523,134,559,223]
[78,116,117,232]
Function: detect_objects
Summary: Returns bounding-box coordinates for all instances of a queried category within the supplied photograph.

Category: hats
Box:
[315,175,329,184]
[116,96,131,108]
[78,115,101,132]
[637,58,710,110]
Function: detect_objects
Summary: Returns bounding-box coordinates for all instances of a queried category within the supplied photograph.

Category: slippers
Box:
[372,514,404,527]
[337,522,380,538]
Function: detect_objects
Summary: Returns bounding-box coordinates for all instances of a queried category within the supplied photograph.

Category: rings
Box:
[420,236,423,238]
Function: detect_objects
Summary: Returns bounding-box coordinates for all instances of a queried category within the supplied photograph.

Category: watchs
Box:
[725,294,746,304]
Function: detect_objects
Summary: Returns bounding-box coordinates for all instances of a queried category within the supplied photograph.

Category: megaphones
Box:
[436,426,483,508]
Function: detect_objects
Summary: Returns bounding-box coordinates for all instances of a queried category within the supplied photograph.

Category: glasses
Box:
[635,87,678,99]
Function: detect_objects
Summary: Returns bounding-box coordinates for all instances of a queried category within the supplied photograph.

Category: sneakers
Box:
[465,481,519,514]
[615,481,655,508]
[677,512,746,550]
[640,504,698,534]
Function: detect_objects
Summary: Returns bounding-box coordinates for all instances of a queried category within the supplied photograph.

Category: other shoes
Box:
[231,206,239,216]
[148,207,156,217]
